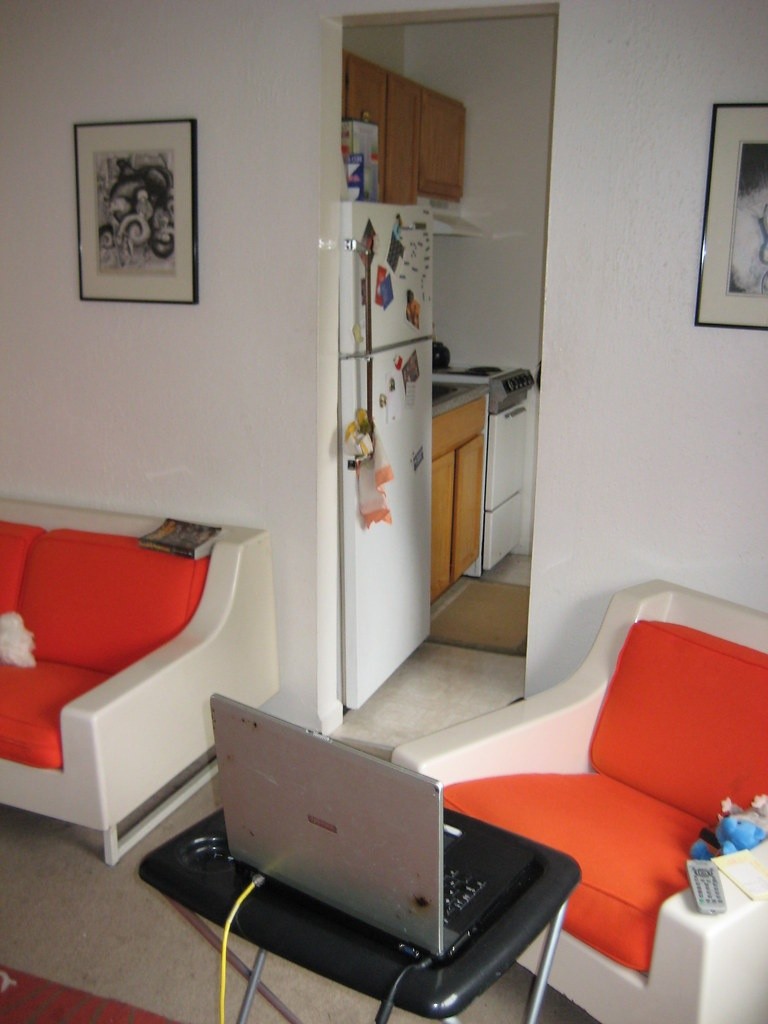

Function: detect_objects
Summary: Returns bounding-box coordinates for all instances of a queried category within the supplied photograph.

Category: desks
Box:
[137,802,582,1024]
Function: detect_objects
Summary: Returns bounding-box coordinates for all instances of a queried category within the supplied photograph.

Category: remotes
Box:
[686,859,727,915]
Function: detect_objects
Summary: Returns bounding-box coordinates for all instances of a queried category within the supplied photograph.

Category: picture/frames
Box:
[74,117,199,306]
[694,101,768,332]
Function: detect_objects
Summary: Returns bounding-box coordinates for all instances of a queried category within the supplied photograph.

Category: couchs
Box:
[391,574,768,1024]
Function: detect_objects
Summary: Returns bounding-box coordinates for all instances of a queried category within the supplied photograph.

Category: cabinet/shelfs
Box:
[341,49,467,206]
[431,396,489,606]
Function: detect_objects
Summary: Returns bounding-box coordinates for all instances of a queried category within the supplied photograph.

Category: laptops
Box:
[210,693,546,972]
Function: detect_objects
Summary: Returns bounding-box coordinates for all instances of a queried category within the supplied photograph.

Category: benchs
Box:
[0,497,275,867]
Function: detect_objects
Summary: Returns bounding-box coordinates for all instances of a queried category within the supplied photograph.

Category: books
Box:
[138,517,223,560]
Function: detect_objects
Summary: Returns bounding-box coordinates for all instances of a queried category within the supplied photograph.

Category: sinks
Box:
[432,383,471,408]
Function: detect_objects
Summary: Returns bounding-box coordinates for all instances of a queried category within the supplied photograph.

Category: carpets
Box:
[421,575,530,657]
